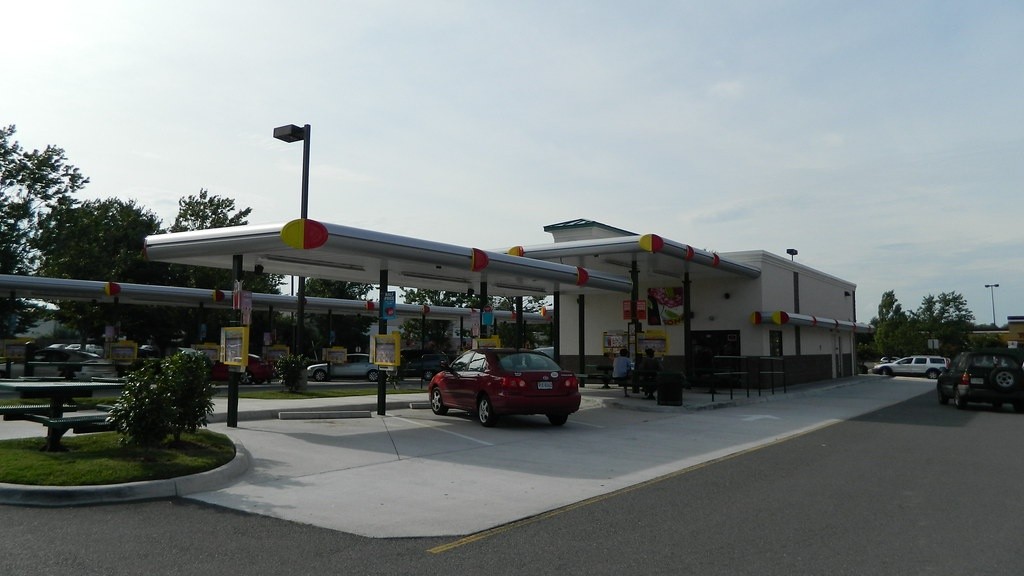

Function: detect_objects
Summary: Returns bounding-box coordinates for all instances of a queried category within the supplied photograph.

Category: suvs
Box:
[872,355,951,379]
[399,348,451,380]
[936,348,1024,410]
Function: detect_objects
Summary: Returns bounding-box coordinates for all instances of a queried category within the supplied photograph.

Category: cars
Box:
[880,357,900,364]
[37,344,158,372]
[307,353,399,382]
[210,354,273,383]
[429,348,582,426]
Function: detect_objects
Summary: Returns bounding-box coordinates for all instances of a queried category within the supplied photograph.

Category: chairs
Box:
[512,359,523,369]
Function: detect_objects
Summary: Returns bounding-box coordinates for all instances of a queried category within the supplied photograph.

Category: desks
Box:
[28,361,112,406]
[0,381,125,452]
[586,365,614,390]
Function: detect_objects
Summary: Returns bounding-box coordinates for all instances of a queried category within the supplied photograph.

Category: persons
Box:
[612,349,631,386]
[397,355,407,381]
[637,348,663,399]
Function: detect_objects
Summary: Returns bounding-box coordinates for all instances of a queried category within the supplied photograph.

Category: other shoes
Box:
[648,395,655,400]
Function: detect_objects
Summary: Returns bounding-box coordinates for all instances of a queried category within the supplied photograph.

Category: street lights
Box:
[272,124,316,391]
[985,283,1001,326]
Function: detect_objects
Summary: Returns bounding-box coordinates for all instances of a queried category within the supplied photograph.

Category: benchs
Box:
[577,374,593,386]
[0,404,114,432]
[91,377,127,382]
[19,376,65,381]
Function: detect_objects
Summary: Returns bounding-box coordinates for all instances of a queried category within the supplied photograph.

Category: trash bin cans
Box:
[656,360,691,408]
